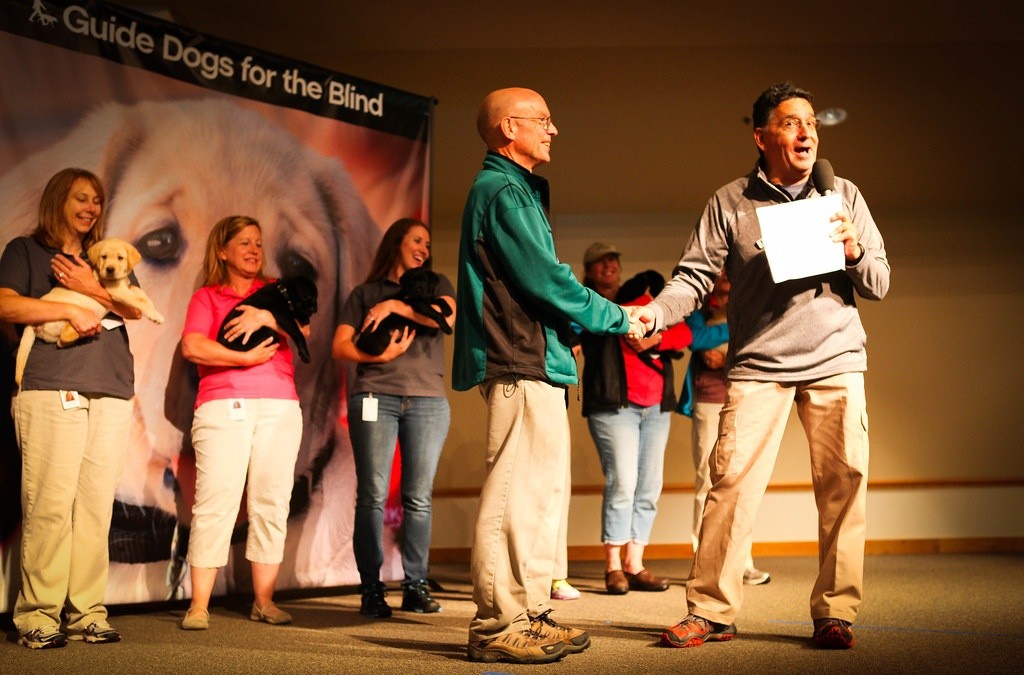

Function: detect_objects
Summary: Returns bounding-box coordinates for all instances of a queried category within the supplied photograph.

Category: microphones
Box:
[811,159,835,196]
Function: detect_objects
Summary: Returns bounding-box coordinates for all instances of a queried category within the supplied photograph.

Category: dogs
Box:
[357,268,453,353]
[15,238,164,384]
[218,275,319,364]
[615,270,666,308]
[0,101,379,608]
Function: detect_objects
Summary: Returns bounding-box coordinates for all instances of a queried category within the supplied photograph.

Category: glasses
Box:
[509,116,550,130]
[764,118,821,132]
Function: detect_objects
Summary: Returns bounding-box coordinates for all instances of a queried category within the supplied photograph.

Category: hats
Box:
[584,242,621,265]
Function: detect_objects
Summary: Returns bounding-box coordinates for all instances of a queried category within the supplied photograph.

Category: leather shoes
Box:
[604,569,630,594]
[622,568,671,591]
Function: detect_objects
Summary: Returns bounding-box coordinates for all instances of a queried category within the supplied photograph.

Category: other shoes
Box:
[182,606,210,630]
[551,578,578,601]
[251,601,291,624]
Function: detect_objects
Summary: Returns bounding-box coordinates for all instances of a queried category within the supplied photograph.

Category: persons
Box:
[331,218,457,618]
[625,80,890,646]
[582,242,692,594]
[182,215,309,629]
[0,168,141,649]
[451,88,646,664]
[550,457,580,600]
[66,392,74,402]
[676,270,770,586]
[234,401,240,408]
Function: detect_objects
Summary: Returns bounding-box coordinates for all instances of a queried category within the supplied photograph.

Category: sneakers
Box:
[68,625,120,642]
[812,617,855,648]
[661,614,737,646]
[18,627,68,649]
[528,609,590,653]
[360,582,392,618]
[400,578,442,612]
[743,567,771,585]
[467,630,570,662]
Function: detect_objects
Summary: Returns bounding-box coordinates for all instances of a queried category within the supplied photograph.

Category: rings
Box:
[371,318,373,320]
[707,360,709,363]
[59,273,63,278]
